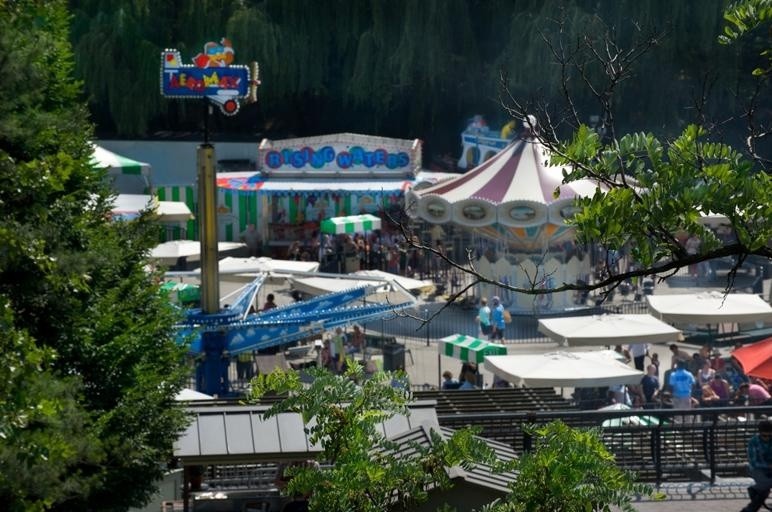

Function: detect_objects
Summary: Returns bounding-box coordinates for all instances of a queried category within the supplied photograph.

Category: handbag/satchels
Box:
[504,310,511,323]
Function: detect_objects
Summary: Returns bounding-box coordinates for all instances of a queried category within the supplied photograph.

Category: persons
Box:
[264,294,275,309]
[322,326,364,372]
[287,230,441,274]
[292,292,302,301]
[442,371,457,387]
[608,344,772,512]
[492,296,506,345]
[476,296,489,341]
[685,235,701,274]
[458,375,476,383]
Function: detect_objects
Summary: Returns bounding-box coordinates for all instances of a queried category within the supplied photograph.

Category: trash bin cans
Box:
[189,331,230,395]
[383,343,405,372]
